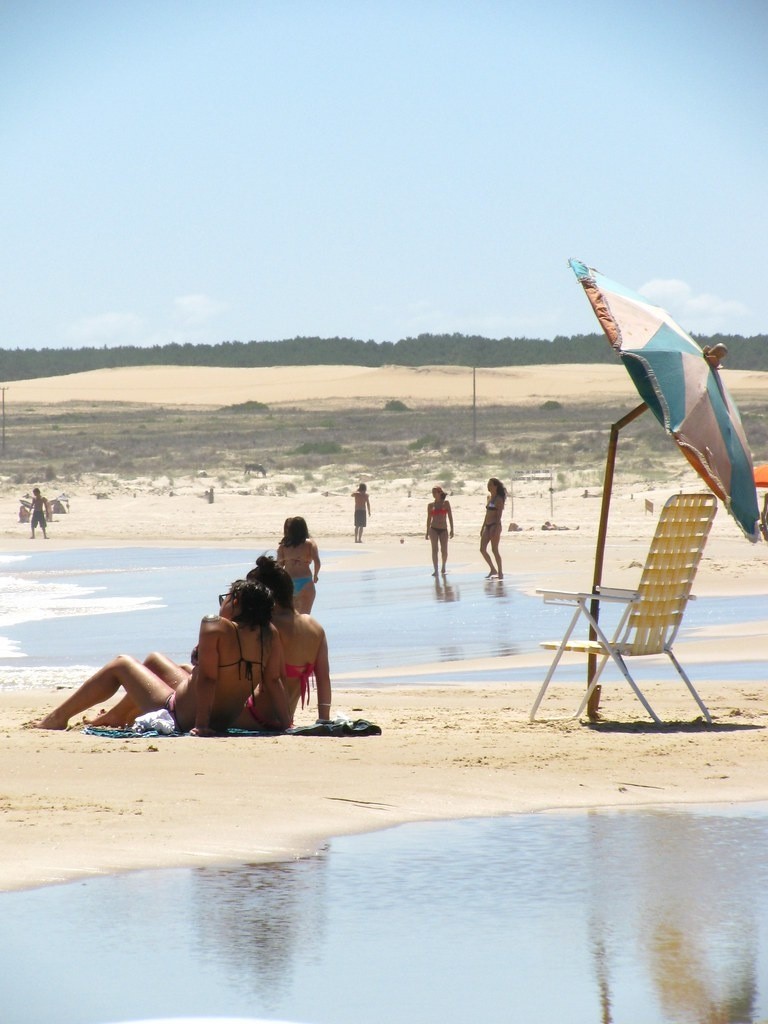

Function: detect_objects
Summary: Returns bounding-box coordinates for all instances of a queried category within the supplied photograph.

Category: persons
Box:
[29,488,47,539]
[19,505,30,523]
[36,517,332,735]
[425,487,454,576]
[209,488,215,504]
[351,483,371,542]
[480,478,509,579]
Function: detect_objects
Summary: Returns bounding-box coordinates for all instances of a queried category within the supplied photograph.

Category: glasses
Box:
[219,592,239,606]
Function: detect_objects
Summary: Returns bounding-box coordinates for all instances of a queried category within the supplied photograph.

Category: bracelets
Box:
[317,703,331,707]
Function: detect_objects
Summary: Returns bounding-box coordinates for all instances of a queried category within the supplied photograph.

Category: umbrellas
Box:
[570,256,762,714]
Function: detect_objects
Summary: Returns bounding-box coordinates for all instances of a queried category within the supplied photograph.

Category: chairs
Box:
[528,494,720,727]
